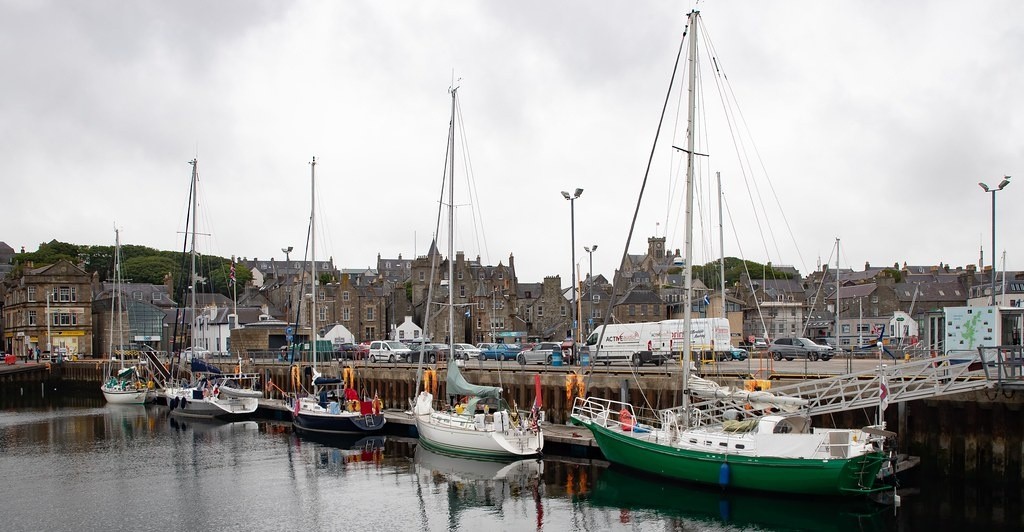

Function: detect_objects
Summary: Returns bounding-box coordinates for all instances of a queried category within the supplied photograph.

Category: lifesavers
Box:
[134,382,142,390]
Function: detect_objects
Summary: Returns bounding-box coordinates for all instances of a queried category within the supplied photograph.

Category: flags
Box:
[228,259,236,288]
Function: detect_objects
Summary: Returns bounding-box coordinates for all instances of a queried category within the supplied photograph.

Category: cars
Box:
[336,340,569,365]
[720,345,748,361]
[186,347,212,359]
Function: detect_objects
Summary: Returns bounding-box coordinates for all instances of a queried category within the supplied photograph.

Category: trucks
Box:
[576,322,672,366]
[661,318,730,364]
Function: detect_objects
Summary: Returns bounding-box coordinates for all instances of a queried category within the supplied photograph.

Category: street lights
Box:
[978,180,1010,306]
[583,245,598,333]
[561,188,584,339]
[282,246,293,350]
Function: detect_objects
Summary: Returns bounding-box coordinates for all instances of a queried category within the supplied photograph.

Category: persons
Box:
[910,335,918,346]
[27,348,33,360]
[36,345,41,364]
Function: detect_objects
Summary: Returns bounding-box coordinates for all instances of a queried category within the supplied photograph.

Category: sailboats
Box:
[100,139,262,418]
[571,467,896,532]
[403,68,544,458]
[259,70,387,432]
[412,443,544,532]
[570,0,900,494]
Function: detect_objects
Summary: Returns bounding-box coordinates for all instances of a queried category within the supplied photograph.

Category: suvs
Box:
[768,337,835,361]
